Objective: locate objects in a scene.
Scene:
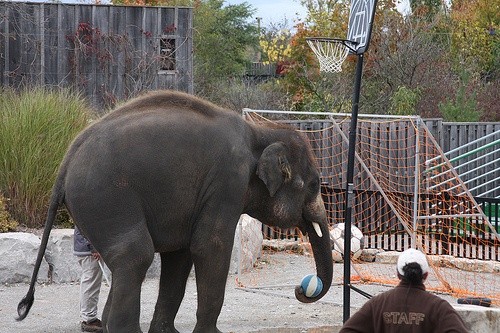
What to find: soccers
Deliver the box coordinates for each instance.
[328,221,365,263]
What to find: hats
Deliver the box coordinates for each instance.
[396,248,430,277]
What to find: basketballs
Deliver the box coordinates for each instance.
[301,275,323,298]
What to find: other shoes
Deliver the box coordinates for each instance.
[81,318,104,333]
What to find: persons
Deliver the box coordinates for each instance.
[73,225,104,333]
[339,249,470,333]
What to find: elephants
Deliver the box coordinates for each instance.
[17,91,333,333]
